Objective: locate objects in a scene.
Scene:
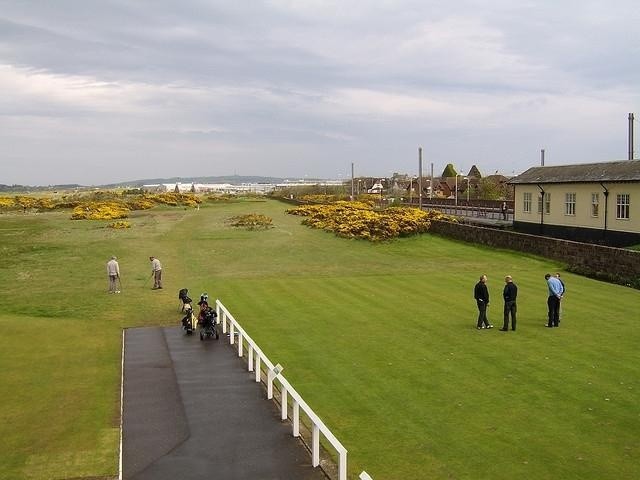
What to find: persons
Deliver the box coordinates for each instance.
[149,256,162,290]
[474,274,494,330]
[106,256,120,293]
[499,275,517,332]
[501,201,509,220]
[544,274,563,328]
[553,273,565,325]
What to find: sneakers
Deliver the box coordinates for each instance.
[486,324,493,329]
[545,323,549,327]
[499,328,506,331]
[478,326,485,329]
[151,287,162,290]
[114,291,120,294]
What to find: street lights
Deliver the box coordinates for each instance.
[357,177,393,202]
[411,176,417,207]
[464,177,469,207]
[426,179,431,204]
[455,173,463,214]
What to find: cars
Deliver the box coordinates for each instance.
[382,196,407,205]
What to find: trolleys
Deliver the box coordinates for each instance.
[200,307,220,340]
